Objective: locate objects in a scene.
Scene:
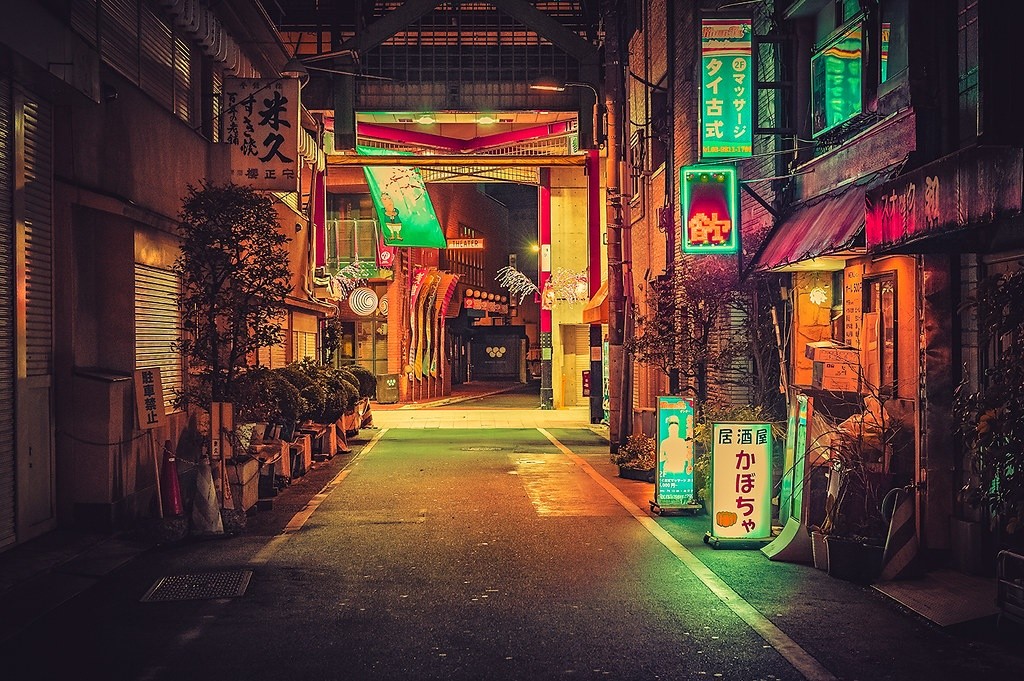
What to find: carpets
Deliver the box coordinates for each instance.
[871,568,1001,627]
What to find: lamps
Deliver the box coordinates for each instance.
[282,54,307,80]
[101,81,121,102]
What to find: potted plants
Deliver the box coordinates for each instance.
[172,355,378,512]
[610,434,656,482]
[809,355,920,578]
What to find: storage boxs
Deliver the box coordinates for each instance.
[811,361,859,392]
[805,339,860,364]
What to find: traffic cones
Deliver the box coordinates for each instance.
[879,486,927,581]
[160,438,184,515]
[188,455,226,538]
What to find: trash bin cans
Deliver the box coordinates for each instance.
[376,374,399,404]
